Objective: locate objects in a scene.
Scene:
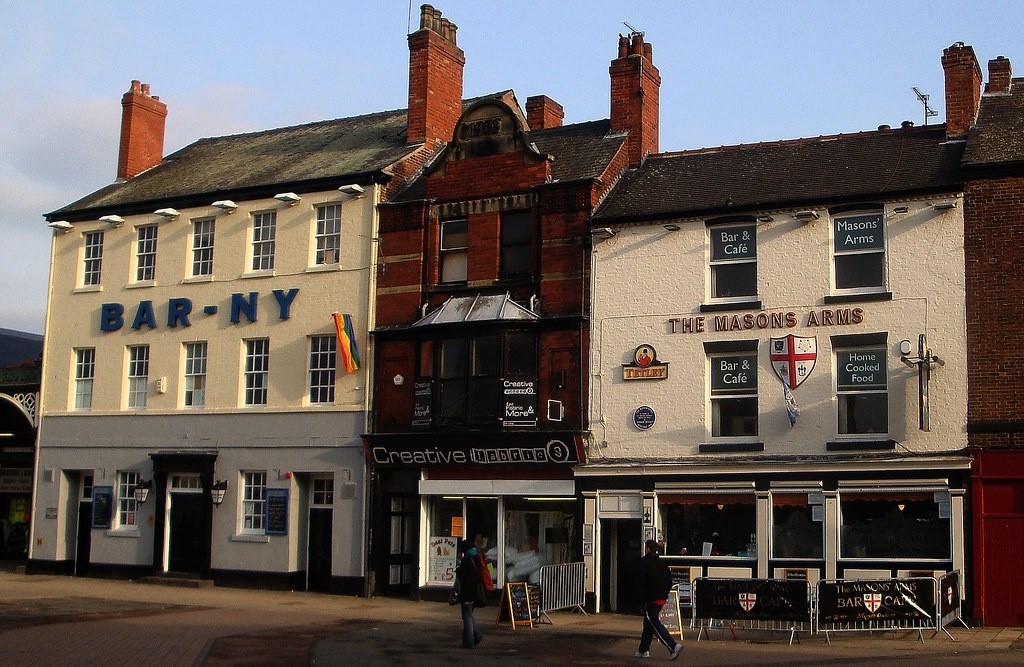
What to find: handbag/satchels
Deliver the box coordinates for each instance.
[478,553,495,590]
[448,578,461,605]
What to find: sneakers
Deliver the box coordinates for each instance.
[670,643,683,661]
[635,650,650,658]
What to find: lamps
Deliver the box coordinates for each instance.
[273,193,301,201]
[154,208,181,218]
[935,201,957,209]
[212,200,239,209]
[663,224,679,231]
[338,184,365,194]
[134,479,152,506]
[48,221,73,229]
[99,214,124,224]
[757,214,772,222]
[209,479,228,508]
[795,211,819,222]
[591,227,614,239]
[894,206,908,213]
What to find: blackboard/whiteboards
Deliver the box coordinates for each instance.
[506,582,532,623]
[667,566,693,605]
[657,590,683,635]
[497,584,513,627]
[784,568,808,584]
[909,570,935,605]
[527,585,540,618]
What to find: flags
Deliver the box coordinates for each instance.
[333,312,361,373]
[781,375,800,428]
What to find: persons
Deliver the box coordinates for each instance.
[633,539,684,661]
[453,539,485,648]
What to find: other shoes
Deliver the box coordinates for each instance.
[475,636,483,645]
